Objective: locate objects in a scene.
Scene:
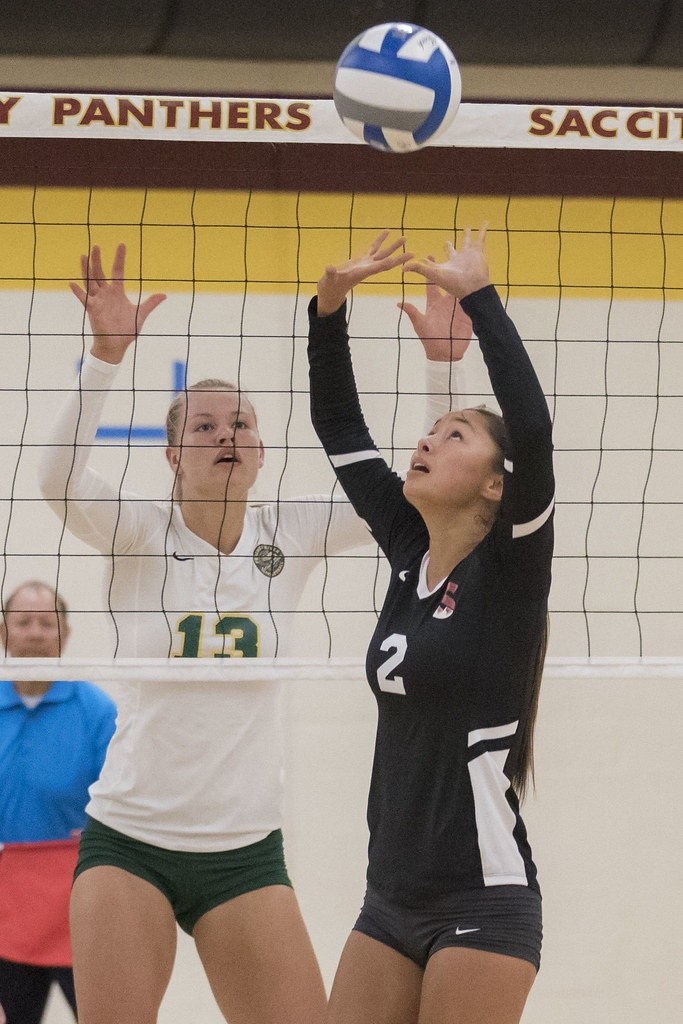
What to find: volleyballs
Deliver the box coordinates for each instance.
[333,20,463,156]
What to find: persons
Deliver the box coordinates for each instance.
[305,225,557,1023]
[36,243,484,1024]
[0,579,120,1024]
